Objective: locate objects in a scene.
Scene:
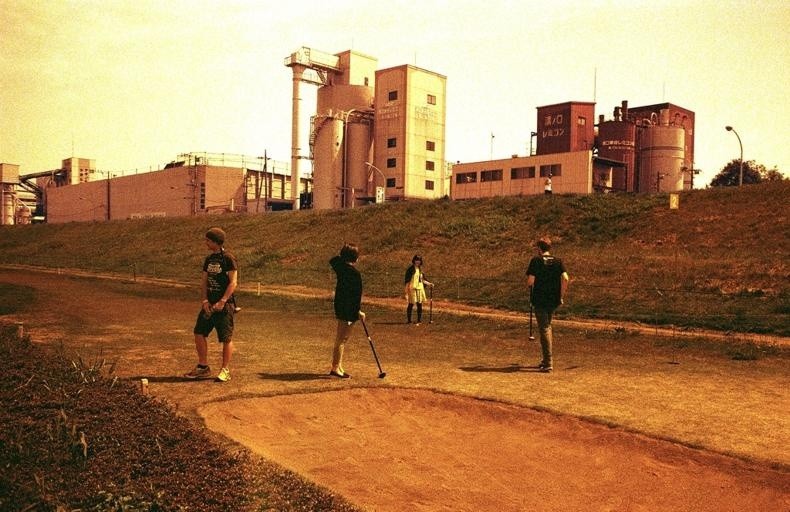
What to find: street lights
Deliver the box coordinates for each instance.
[79,195,97,222]
[364,162,387,203]
[726,125,744,187]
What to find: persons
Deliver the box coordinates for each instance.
[328,240,366,381]
[403,254,435,325]
[183,227,238,382]
[544,173,553,194]
[524,234,570,372]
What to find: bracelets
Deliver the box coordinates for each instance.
[220,297,227,303]
[201,299,209,303]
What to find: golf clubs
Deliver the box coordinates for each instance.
[221,247,241,313]
[359,317,384,377]
[527,286,534,340]
[429,288,433,323]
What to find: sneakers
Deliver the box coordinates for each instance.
[540,363,553,371]
[184,365,213,380]
[329,370,351,380]
[343,372,351,376]
[215,367,232,382]
